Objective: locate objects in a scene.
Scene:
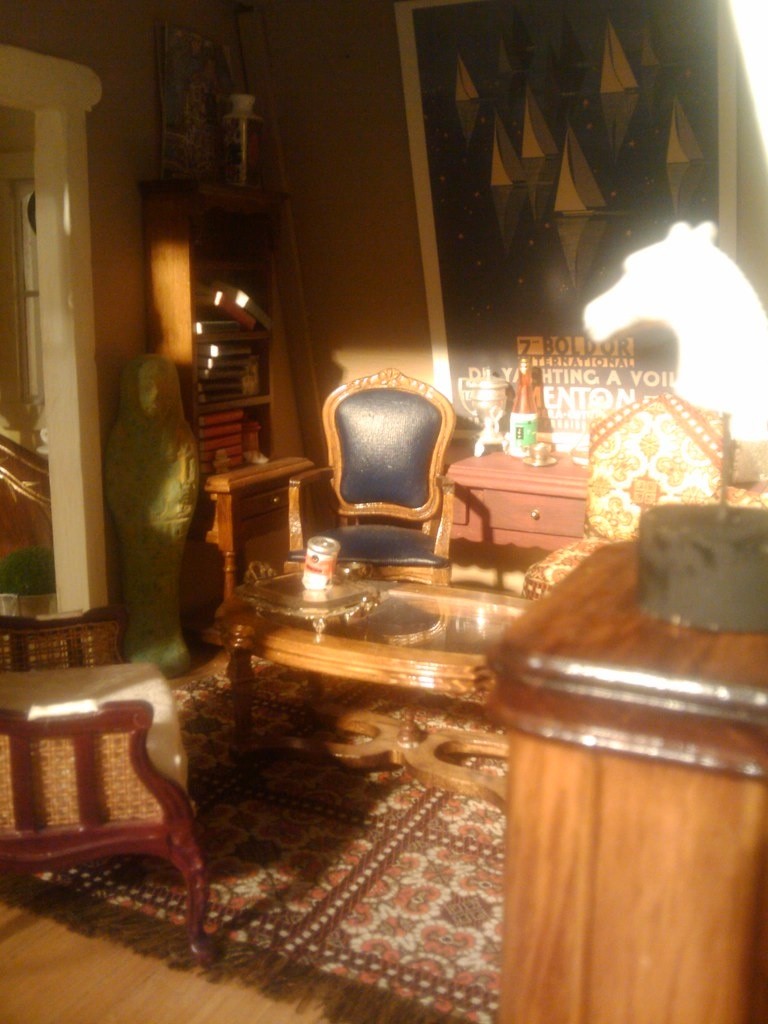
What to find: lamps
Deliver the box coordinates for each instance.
[223,91,267,184]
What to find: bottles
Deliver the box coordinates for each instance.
[224,94,265,189]
[507,357,537,458]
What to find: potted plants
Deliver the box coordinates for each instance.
[0,543,58,619]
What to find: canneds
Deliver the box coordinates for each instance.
[301,536,341,591]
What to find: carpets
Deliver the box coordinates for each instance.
[0,638,506,1023]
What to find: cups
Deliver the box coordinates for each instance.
[531,442,552,462]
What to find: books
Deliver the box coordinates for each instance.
[188,280,272,473]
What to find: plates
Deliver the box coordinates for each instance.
[522,457,557,467]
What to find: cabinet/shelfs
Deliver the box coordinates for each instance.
[140,178,286,469]
[485,545,768,1023]
[192,456,314,598]
[446,453,590,551]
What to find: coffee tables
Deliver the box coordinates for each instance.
[220,580,535,813]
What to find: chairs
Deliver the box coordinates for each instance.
[520,395,727,600]
[0,604,219,962]
[285,368,457,584]
